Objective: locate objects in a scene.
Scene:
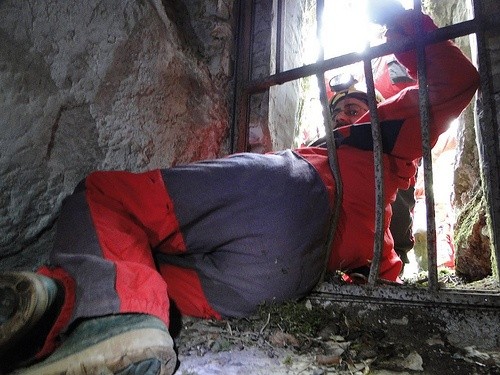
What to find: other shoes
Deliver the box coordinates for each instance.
[9,313,177,375]
[0,270,60,354]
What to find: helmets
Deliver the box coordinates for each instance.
[331,83,385,109]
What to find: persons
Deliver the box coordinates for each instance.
[321,26,423,280]
[1,1,484,375]
